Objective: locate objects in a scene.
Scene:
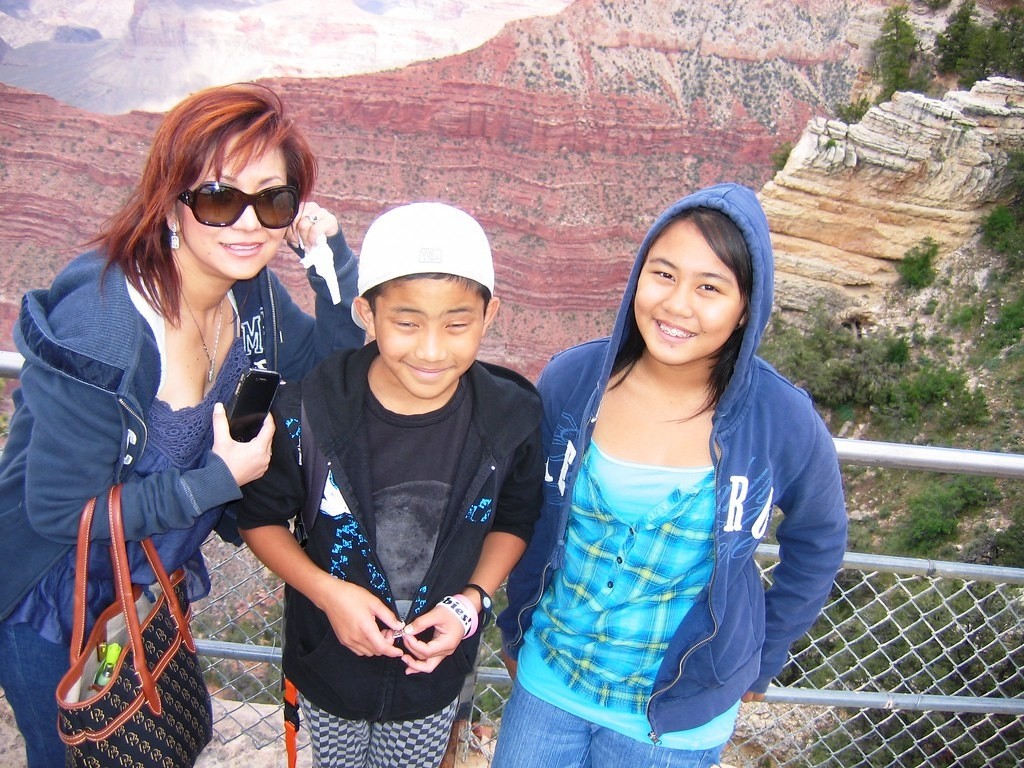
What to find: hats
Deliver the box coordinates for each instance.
[351,203,495,331]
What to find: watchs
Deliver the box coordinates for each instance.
[465,584,493,635]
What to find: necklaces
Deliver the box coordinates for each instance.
[178,284,224,382]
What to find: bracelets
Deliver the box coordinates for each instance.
[437,594,478,639]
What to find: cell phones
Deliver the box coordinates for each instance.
[226,369,282,442]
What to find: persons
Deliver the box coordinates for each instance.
[232,204,544,768]
[491,183,847,768]
[0,82,365,768]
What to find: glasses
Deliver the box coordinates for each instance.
[177,176,301,229]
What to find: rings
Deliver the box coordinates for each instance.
[302,213,319,223]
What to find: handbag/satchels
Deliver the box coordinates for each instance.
[56,484,213,768]
